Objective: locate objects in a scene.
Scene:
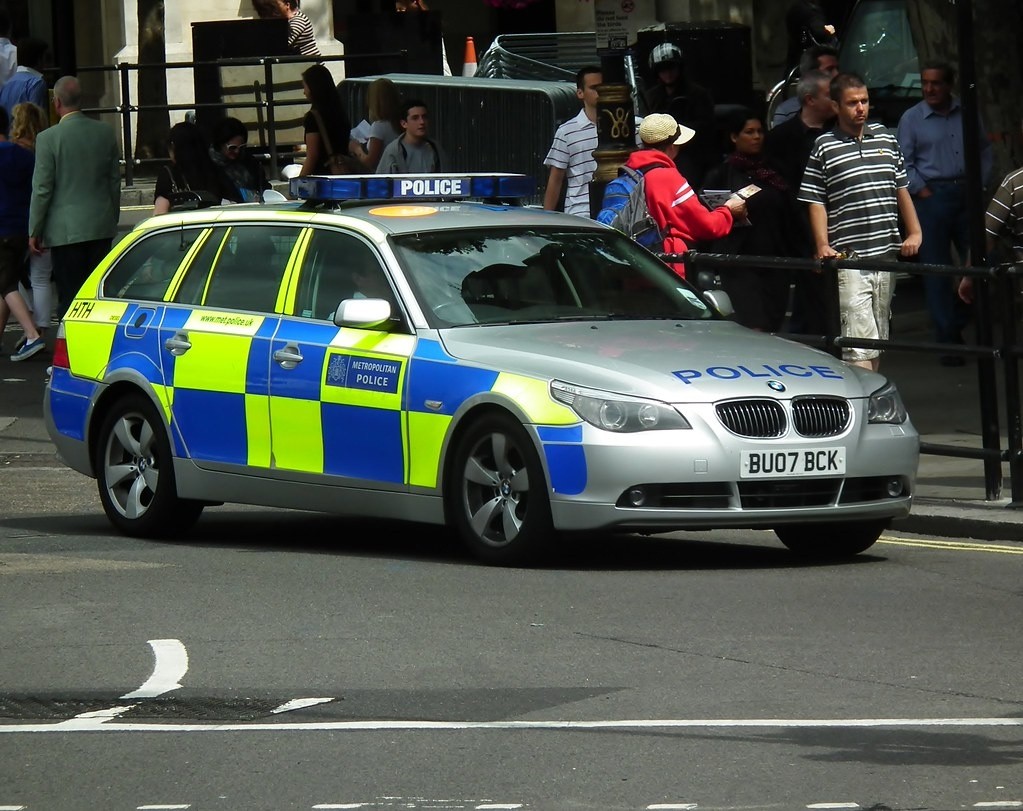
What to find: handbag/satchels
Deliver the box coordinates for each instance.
[329,154,374,175]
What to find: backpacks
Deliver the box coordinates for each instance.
[596,164,671,253]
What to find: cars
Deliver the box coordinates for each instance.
[39,174,922,570]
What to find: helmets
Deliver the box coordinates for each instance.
[647,42,685,70]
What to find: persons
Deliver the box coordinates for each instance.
[0,106,46,362]
[958,167,1023,304]
[697,44,839,357]
[10,103,58,355]
[0,8,49,128]
[153,117,272,215]
[375,99,448,174]
[625,113,748,281]
[796,73,922,372]
[396,0,428,11]
[895,60,996,367]
[28,76,121,375]
[286,65,351,200]
[325,245,395,331]
[251,0,325,65]
[348,78,407,175]
[639,42,723,192]
[543,68,644,221]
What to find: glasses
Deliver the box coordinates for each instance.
[225,143,247,151]
[51,97,59,103]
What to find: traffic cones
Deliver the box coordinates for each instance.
[462,35,478,78]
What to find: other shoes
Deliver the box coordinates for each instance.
[10,337,45,361]
[941,337,965,366]
[15,328,41,349]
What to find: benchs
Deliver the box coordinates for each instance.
[134,258,275,309]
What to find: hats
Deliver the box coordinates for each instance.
[640,112,696,149]
